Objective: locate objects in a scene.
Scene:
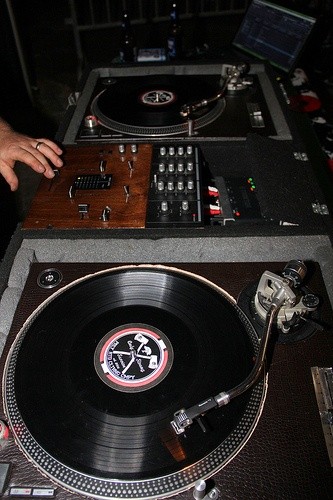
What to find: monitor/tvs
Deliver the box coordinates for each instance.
[230,0,316,73]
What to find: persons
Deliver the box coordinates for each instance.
[0,118,64,192]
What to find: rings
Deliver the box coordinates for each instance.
[36,142,43,150]
